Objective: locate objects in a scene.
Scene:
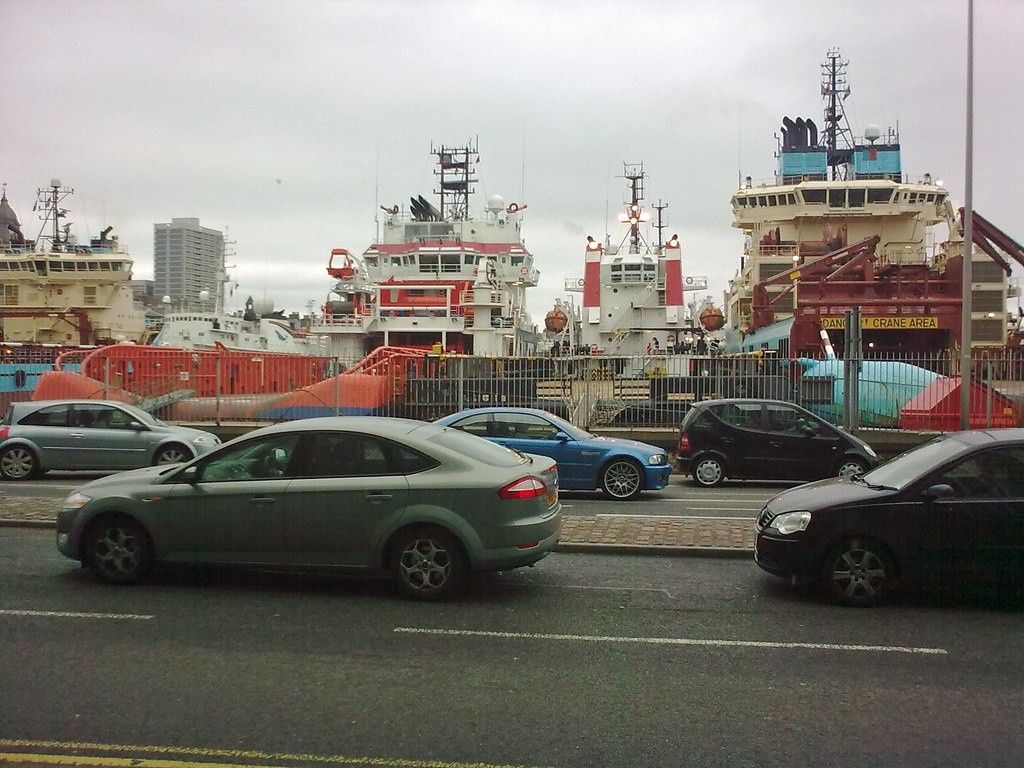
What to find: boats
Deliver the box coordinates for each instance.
[0,181,323,402]
[546,310,568,331]
[309,135,541,374]
[715,46,1024,386]
[323,249,355,278]
[699,304,724,332]
[541,162,725,398]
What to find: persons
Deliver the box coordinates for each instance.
[96,409,113,428]
[653,337,660,350]
[647,343,651,356]
[514,418,532,439]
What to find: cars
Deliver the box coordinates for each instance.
[57,417,562,599]
[0,397,226,479]
[675,398,881,486]
[429,407,673,501]
[752,426,1024,608]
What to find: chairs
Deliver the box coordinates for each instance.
[379,443,414,473]
[332,437,363,475]
[500,421,510,436]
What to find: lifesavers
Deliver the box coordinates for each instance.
[393,205,399,212]
[67,334,72,339]
[15,370,26,386]
[590,343,598,350]
[510,203,518,212]
[578,278,584,285]
[56,288,62,294]
[520,267,528,275]
[667,335,674,341]
[686,276,693,284]
